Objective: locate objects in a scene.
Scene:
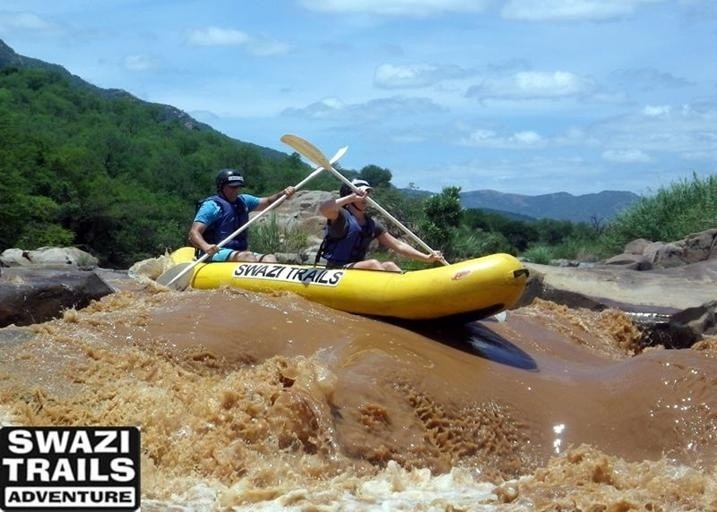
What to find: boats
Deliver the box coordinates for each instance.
[168,244,534,325]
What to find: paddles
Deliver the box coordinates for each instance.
[155,146,350,291]
[281,134,451,267]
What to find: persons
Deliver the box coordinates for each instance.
[313,178,445,274]
[187,168,295,262]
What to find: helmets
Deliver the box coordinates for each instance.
[216,168,246,190]
[341,177,374,197]
